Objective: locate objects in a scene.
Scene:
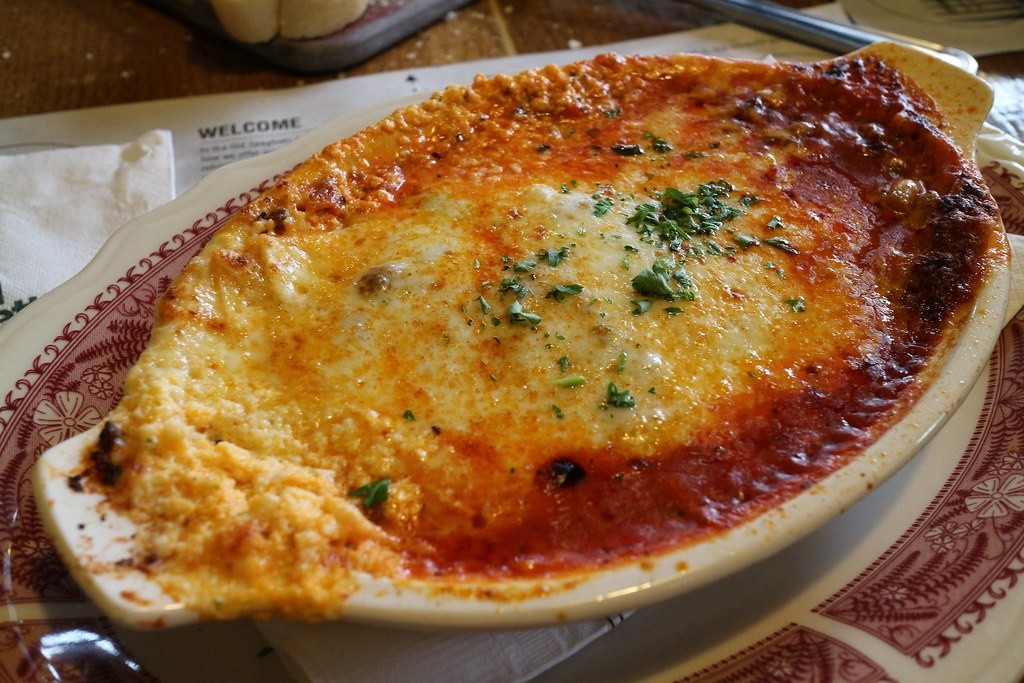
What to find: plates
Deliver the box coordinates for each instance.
[0,100,1024,683]
[32,41,1010,633]
